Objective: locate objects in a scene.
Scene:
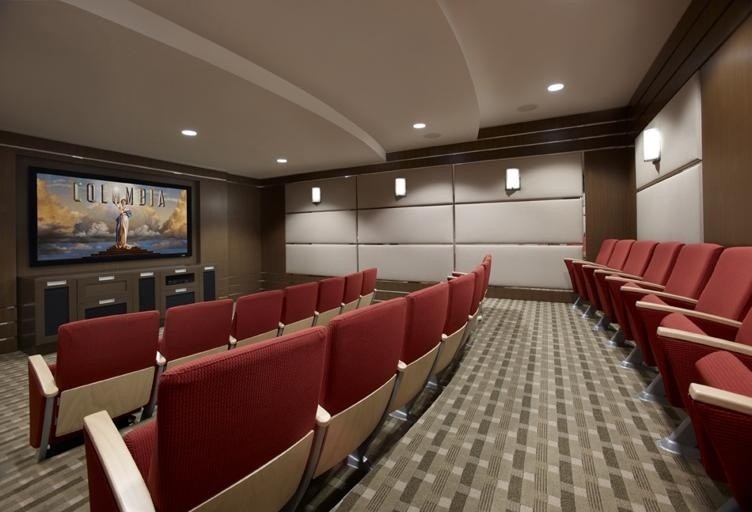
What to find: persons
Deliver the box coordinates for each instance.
[111,196,135,250]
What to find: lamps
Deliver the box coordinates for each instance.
[642,128,662,162]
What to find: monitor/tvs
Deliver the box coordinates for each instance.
[27,167,192,267]
